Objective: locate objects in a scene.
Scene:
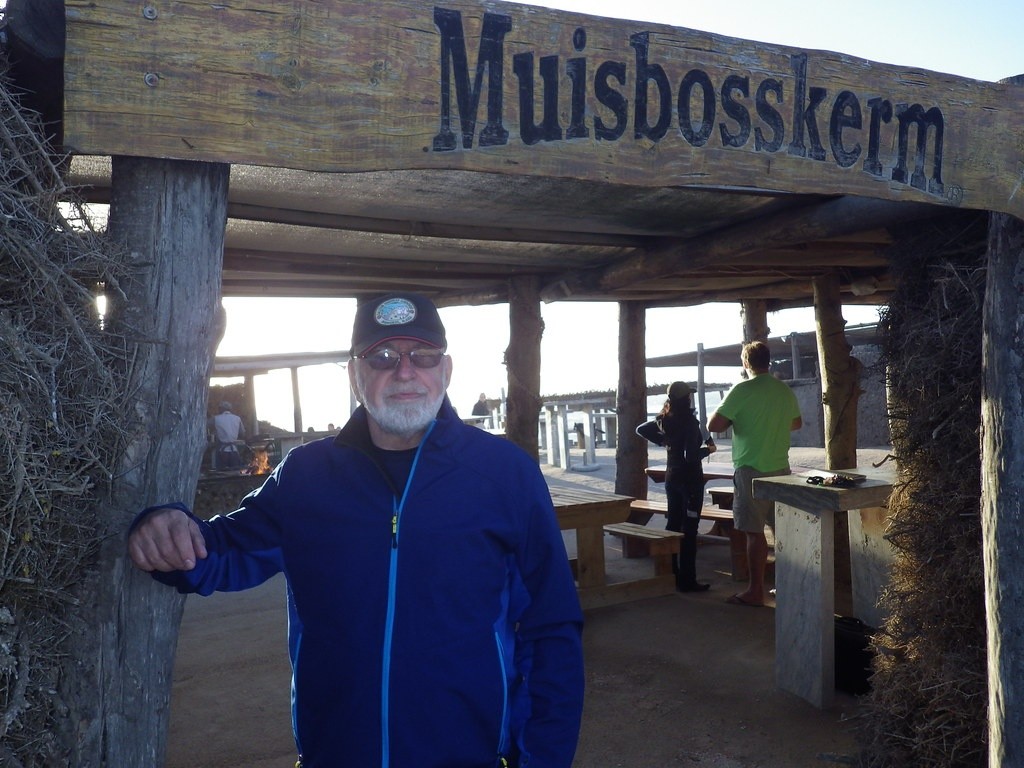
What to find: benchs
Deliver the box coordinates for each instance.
[602,523,684,577]
[704,485,734,537]
[628,500,749,581]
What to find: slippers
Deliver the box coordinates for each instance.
[726,593,766,606]
[769,589,776,598]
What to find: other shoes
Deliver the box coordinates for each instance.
[672,568,678,576]
[677,583,710,593]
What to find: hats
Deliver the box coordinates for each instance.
[669,382,697,401]
[351,291,447,359]
[223,402,232,411]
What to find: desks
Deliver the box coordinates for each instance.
[644,464,775,576]
[751,465,895,710]
[549,483,675,611]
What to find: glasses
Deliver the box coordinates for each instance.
[352,347,444,371]
[806,476,826,485]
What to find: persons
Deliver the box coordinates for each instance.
[472,393,489,429]
[307,427,314,432]
[635,382,716,593]
[206,401,245,471]
[328,423,338,431]
[706,340,803,604]
[128,292,583,768]
